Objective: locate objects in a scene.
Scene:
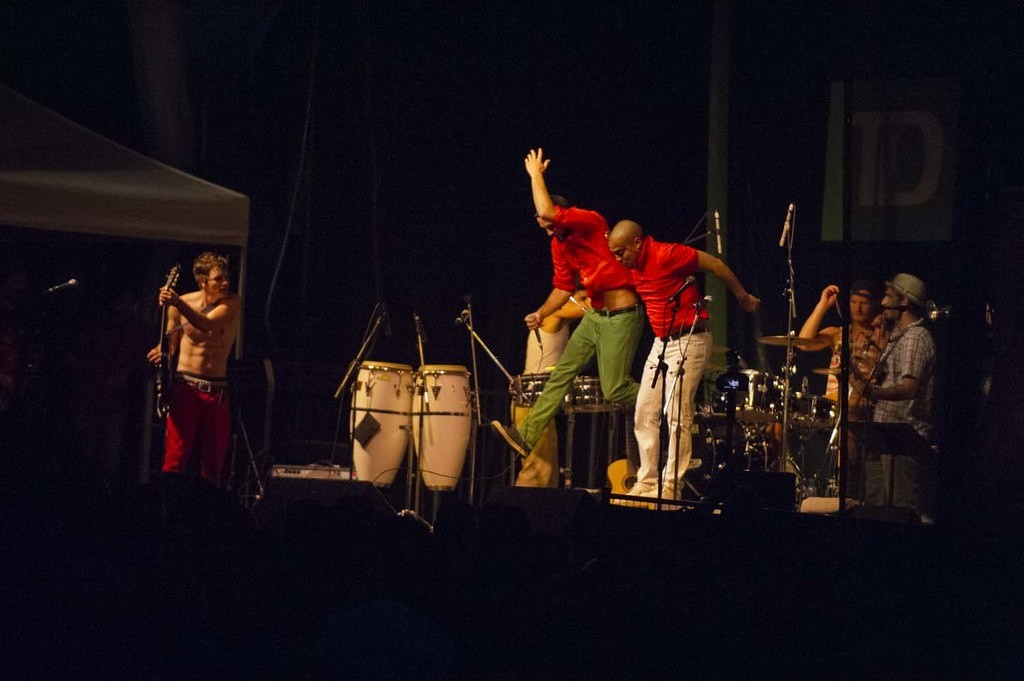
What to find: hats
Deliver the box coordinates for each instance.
[850,281,877,299]
[885,272,930,308]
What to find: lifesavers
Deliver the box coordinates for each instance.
[509,373,552,407]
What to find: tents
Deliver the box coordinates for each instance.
[0,85,250,357]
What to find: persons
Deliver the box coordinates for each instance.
[510,288,589,490]
[796,273,890,501]
[147,253,240,488]
[490,147,642,456]
[850,273,937,519]
[0,398,1024,681]
[606,219,760,500]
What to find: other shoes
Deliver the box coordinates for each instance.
[490,419,530,460]
[614,483,654,503]
[640,485,683,501]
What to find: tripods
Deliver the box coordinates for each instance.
[759,232,815,497]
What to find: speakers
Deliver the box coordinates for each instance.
[716,470,798,516]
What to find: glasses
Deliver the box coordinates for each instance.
[199,272,231,282]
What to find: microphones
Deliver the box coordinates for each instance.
[415,312,429,342]
[714,211,722,255]
[779,203,793,247]
[529,315,543,349]
[380,304,394,337]
[48,277,77,293]
[669,276,696,301]
[454,309,468,325]
[693,294,714,308]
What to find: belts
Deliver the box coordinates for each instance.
[595,303,643,316]
[176,374,230,393]
[661,326,711,343]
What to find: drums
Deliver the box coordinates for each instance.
[745,420,783,458]
[730,364,786,423]
[410,365,472,491]
[789,392,834,429]
[562,377,621,415]
[349,361,415,487]
[704,409,738,446]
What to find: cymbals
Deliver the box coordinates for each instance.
[706,363,722,371]
[758,334,825,346]
[713,345,730,353]
[812,369,841,375]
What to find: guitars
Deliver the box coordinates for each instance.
[156,265,180,419]
